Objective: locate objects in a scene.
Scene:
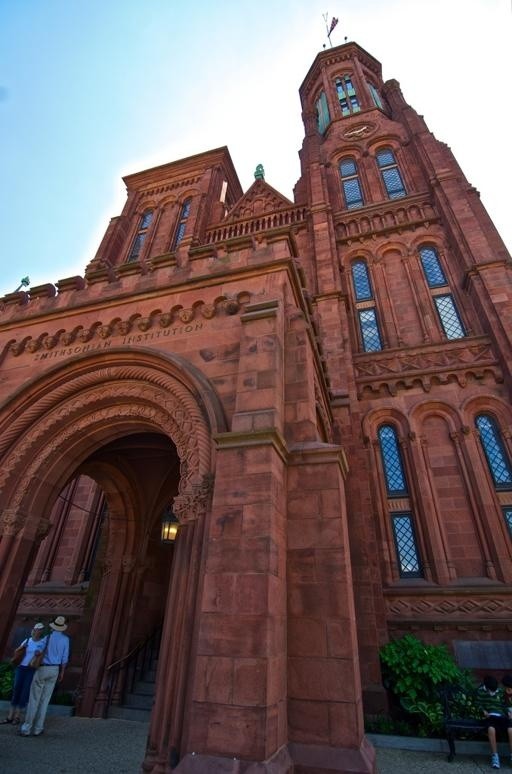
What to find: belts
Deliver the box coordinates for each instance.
[40,663,60,666]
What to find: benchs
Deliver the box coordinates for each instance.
[441,686,510,763]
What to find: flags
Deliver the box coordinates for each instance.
[328,15,339,37]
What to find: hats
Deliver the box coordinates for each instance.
[49,614,69,631]
[34,622,45,630]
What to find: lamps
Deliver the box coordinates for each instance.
[160,507,180,544]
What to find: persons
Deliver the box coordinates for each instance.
[0,621,46,725]
[500,673,511,722]
[15,615,70,737]
[473,673,512,769]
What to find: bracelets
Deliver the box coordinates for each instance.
[61,669,65,672]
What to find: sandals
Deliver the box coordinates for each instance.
[0,716,45,737]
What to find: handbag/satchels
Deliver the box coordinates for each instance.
[10,637,30,666]
[29,647,46,670]
